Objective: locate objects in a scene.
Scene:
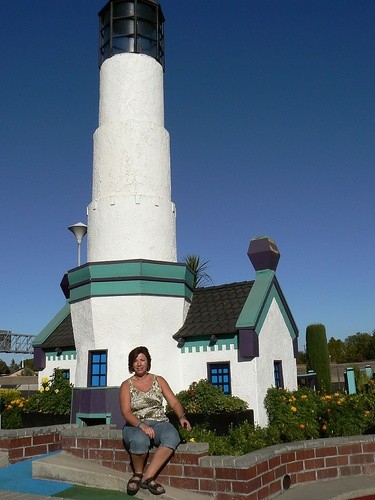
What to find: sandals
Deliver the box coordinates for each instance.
[126,473,143,496]
[139,476,165,495]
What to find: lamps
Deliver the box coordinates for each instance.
[68,221,88,267]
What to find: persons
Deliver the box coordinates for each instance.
[119,347,192,495]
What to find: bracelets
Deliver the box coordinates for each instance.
[137,421,144,428]
[177,415,187,422]
